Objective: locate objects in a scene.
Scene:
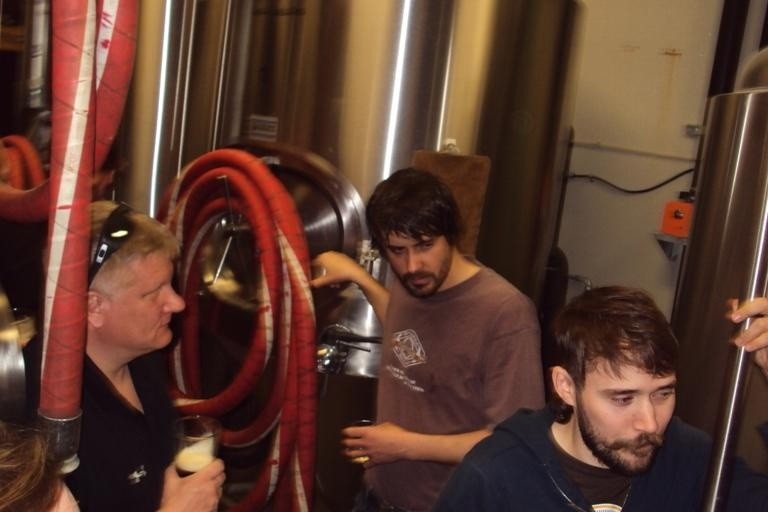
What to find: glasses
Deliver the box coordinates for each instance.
[89,200,136,288]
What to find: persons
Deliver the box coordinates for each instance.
[308,170,547,512]
[64,199,227,512]
[0,422,81,512]
[434,286,768,512]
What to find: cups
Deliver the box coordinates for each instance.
[175,415,218,477]
